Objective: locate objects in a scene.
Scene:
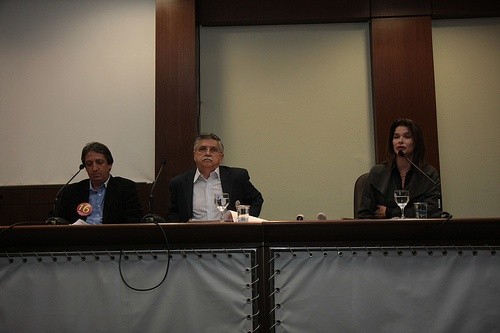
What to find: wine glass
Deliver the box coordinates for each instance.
[214,194,229,223]
[394,190,409,219]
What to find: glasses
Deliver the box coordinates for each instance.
[198,145,220,154]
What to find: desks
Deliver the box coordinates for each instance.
[263,217,500,333]
[0,222,265,333]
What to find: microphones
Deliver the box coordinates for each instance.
[46,163,84,225]
[398,150,438,186]
[141,159,167,223]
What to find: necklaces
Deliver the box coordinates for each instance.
[401,175,406,177]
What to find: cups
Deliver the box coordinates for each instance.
[236,205,250,222]
[413,203,427,219]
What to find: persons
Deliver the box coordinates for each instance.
[357,118,442,218]
[46,142,143,224]
[165,132,264,223]
